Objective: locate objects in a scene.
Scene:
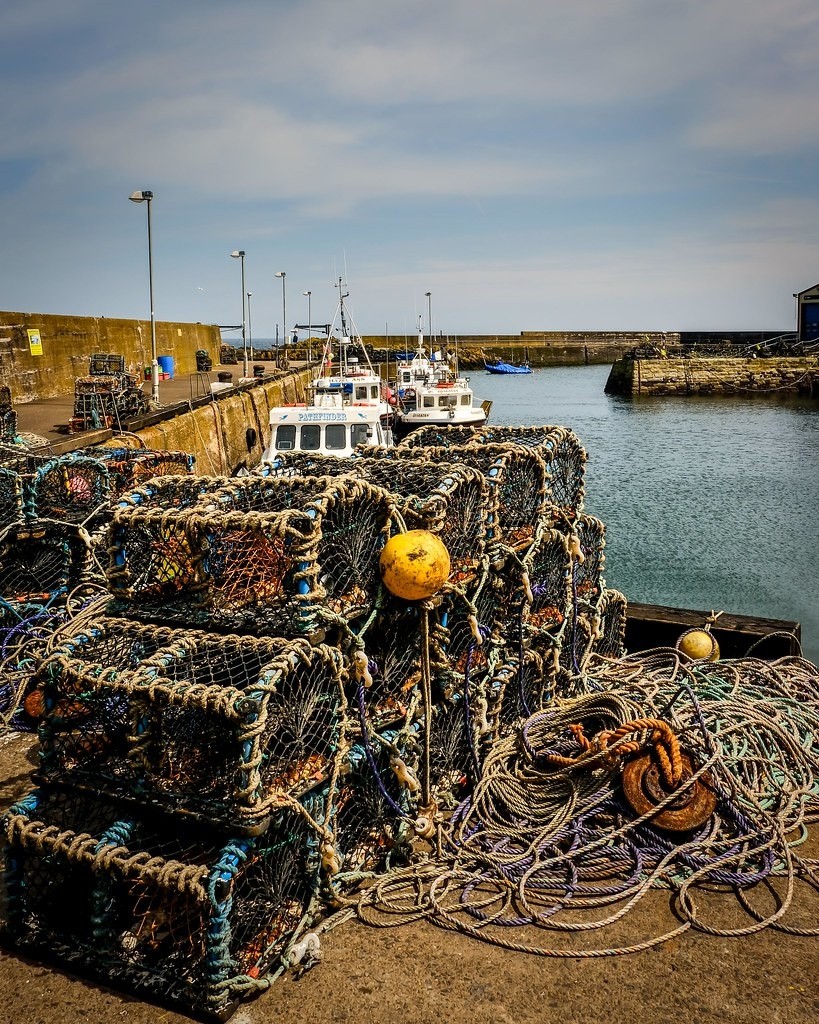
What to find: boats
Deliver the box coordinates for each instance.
[236,252,494,477]
[480,350,534,374]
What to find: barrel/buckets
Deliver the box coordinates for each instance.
[157,355,174,379]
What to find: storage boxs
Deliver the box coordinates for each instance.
[164,373,170,380]
[145,375,150,380]
[149,374,163,380]
[145,366,163,375]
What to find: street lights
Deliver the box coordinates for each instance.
[301,291,312,362]
[246,291,253,362]
[230,250,249,378]
[127,190,160,407]
[275,271,287,360]
[425,293,433,359]
